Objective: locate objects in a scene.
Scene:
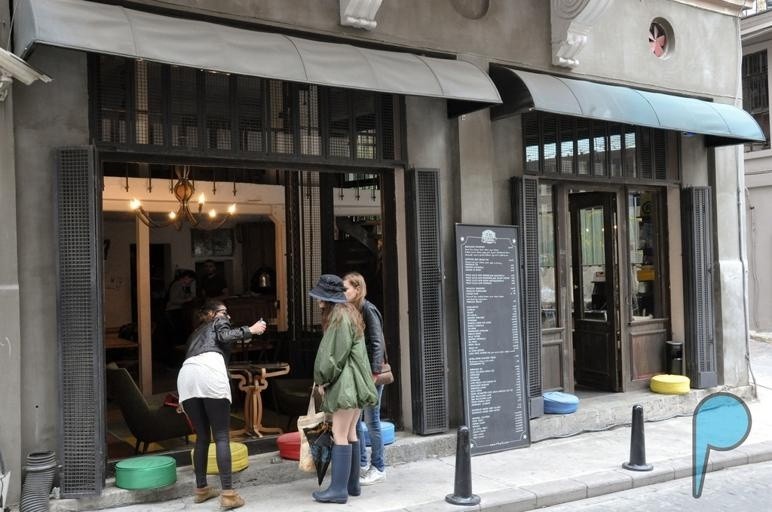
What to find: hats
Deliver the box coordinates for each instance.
[308,274,347,303]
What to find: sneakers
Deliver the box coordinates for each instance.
[359,465,385,486]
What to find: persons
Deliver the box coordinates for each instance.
[176,296,267,511]
[310,274,380,505]
[340,271,396,486]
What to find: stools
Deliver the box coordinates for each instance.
[650,374,692,395]
[275,432,306,461]
[190,442,248,474]
[361,421,396,446]
[542,392,579,413]
[115,455,176,490]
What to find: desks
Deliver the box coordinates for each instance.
[226,360,290,440]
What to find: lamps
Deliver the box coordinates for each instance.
[129,164,237,231]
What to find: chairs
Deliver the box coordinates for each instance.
[271,376,318,413]
[106,361,187,455]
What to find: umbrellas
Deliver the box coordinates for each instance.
[303,422,335,487]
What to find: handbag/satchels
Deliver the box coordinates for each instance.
[374,364,394,385]
[297,410,326,474]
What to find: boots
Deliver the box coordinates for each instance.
[312,443,352,504]
[220,489,244,508]
[349,439,362,496]
[194,486,218,504]
